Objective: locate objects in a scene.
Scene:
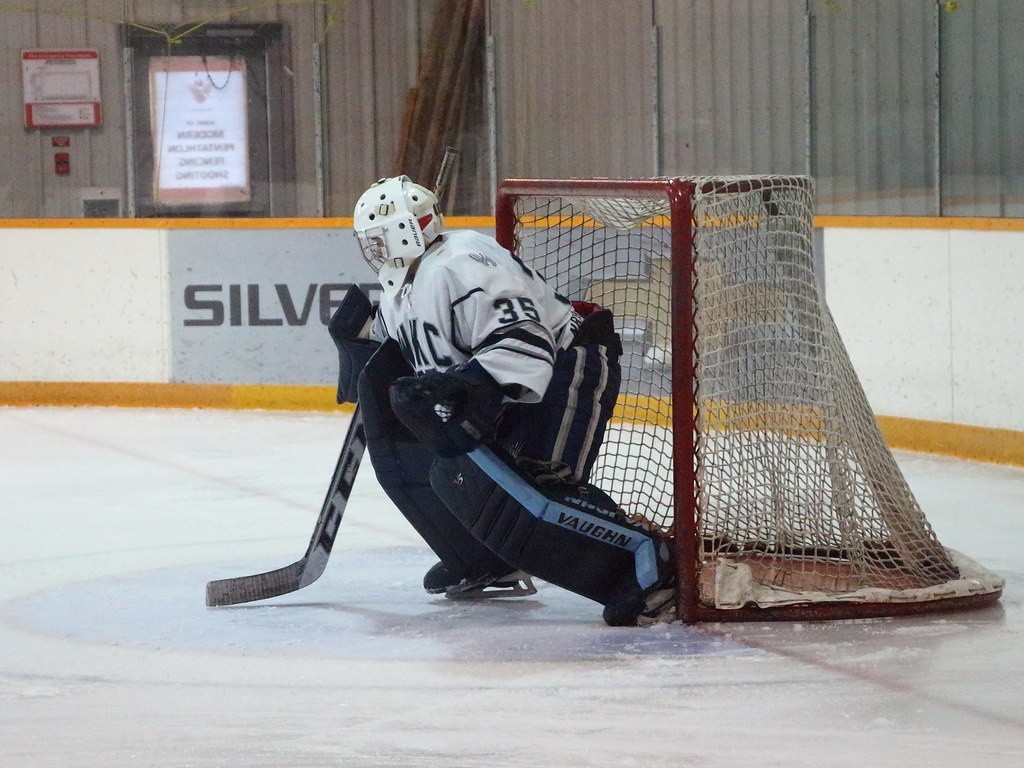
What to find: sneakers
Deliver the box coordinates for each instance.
[603,537,677,627]
[424,561,538,597]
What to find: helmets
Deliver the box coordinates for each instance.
[354,175,444,300]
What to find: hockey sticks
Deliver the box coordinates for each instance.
[205,146,460,608]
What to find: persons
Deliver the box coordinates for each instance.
[352,174,678,626]
